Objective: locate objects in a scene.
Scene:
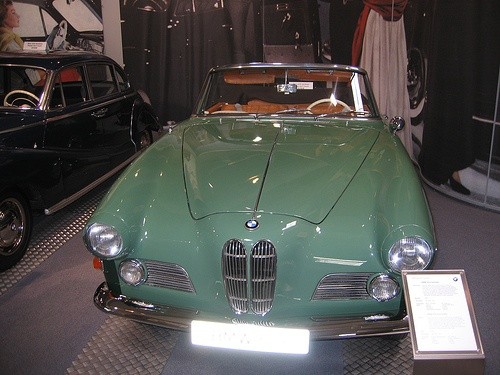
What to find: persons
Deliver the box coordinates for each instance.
[0,0,24,52]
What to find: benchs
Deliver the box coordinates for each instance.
[216,96,371,118]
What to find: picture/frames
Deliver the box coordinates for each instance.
[401,269,486,361]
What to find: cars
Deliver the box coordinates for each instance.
[1,49,168,275]
[84,62,437,341]
